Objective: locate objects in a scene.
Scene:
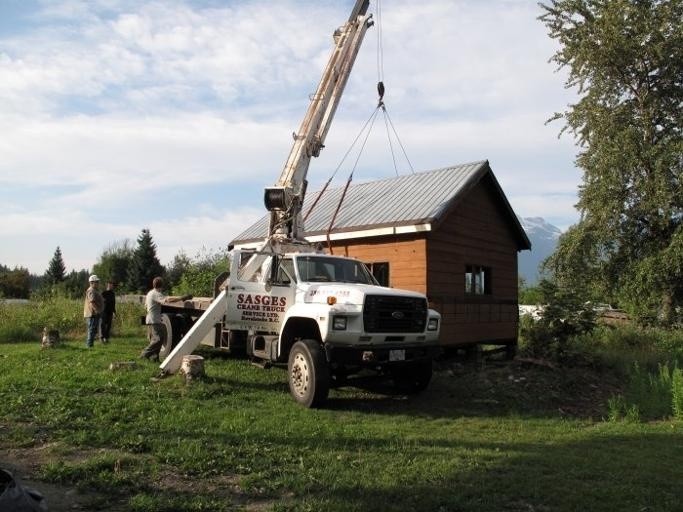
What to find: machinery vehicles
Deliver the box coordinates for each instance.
[138,0,441,412]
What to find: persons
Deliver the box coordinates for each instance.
[84,275,105,347]
[99,282,115,342]
[139,276,193,362]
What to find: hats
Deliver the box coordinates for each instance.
[89,275,99,282]
